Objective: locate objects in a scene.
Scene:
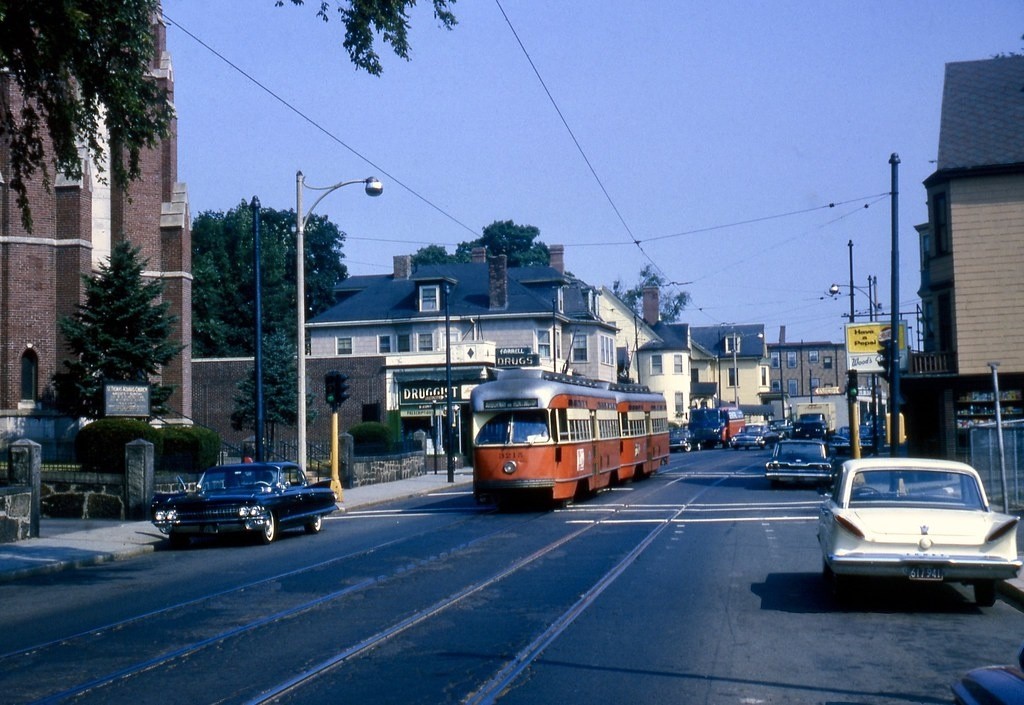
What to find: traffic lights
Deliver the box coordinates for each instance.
[339,376,351,406]
[846,371,859,396]
[324,373,339,405]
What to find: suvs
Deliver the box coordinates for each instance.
[764,420,790,445]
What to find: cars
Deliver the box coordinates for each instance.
[948,646,1024,705]
[818,456,1020,607]
[153,462,336,546]
[670,429,701,450]
[730,424,770,450]
[765,440,833,488]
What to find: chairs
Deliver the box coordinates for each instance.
[255,471,273,487]
[224,473,247,488]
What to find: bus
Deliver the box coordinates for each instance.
[469,369,669,507]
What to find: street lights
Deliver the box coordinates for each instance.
[249,193,269,461]
[732,331,764,406]
[293,170,383,481]
[442,285,457,482]
[885,152,902,455]
[829,276,884,454]
[847,239,856,450]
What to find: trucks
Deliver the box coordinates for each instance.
[689,406,745,449]
[794,403,835,439]
